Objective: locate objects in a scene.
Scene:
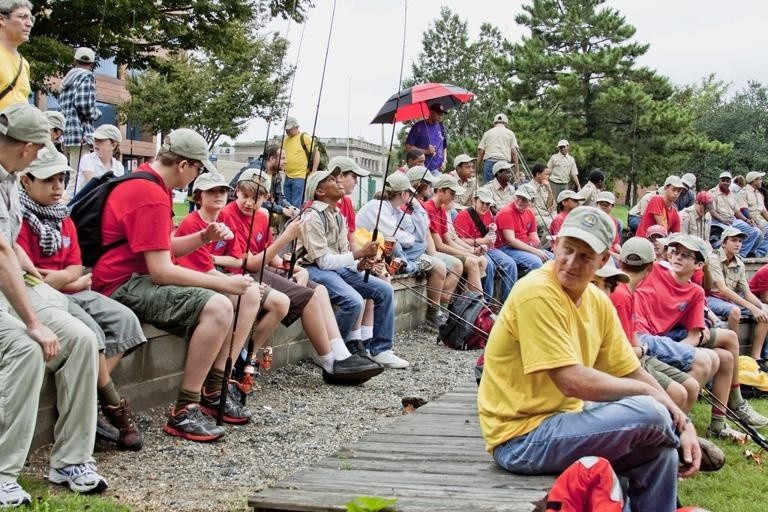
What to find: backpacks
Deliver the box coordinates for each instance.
[65,170,175,267]
[300,132,330,171]
[437,290,495,351]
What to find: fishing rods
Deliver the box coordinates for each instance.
[216,2,337,427]
[697,142,707,240]
[129,2,134,172]
[379,79,477,260]
[500,142,559,245]
[394,212,768,458]
[364,2,411,282]
[70,2,109,200]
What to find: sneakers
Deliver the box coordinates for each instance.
[49,462,109,493]
[162,404,225,440]
[197,385,252,421]
[306,338,409,385]
[0,481,32,508]
[706,422,752,446]
[722,401,768,430]
[681,438,725,471]
[424,309,448,329]
[95,398,143,452]
[414,258,434,272]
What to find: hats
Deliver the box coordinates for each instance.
[162,127,219,177]
[665,173,696,190]
[0,102,77,180]
[514,183,536,201]
[746,171,765,182]
[191,169,273,194]
[303,156,372,201]
[594,255,631,284]
[696,191,715,211]
[471,187,496,204]
[556,190,616,254]
[453,154,478,169]
[386,165,467,195]
[721,228,747,244]
[285,116,299,130]
[429,103,447,115]
[719,172,732,179]
[494,114,508,124]
[74,47,95,62]
[619,225,709,266]
[556,140,569,148]
[85,124,122,143]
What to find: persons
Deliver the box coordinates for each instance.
[58,47,102,197]
[0,0,36,111]
[0,103,768,512]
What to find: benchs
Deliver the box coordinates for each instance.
[252,378,561,512]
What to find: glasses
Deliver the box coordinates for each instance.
[7,12,36,24]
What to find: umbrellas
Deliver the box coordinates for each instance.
[369,83,475,145]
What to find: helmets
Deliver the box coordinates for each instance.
[492,161,516,177]
